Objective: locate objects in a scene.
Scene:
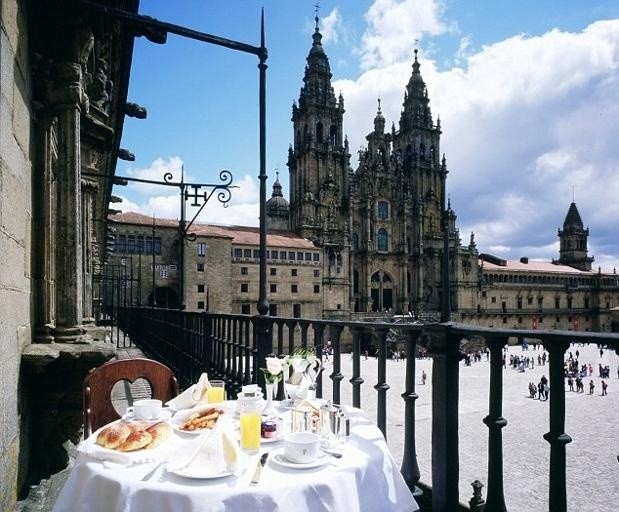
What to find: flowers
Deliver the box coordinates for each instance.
[258,343,325,384]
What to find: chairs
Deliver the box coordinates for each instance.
[82,357,180,440]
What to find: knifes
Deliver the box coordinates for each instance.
[250,453,268,484]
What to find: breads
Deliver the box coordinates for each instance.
[97,419,170,452]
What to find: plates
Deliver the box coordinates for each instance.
[270,447,329,468]
[123,409,171,422]
[76,420,181,462]
[174,404,228,434]
[170,448,247,480]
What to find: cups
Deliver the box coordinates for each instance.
[283,432,330,463]
[240,404,261,453]
[207,379,225,404]
[127,399,162,419]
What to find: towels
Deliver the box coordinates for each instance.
[296,399,328,411]
[165,424,248,476]
[165,371,211,411]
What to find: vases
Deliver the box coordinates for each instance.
[261,383,279,416]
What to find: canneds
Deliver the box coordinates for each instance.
[261,420,278,438]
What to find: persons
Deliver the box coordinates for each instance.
[322,342,334,362]
[458,338,618,400]
[421,370,426,385]
[363,351,369,360]
[389,348,432,362]
[374,348,379,359]
